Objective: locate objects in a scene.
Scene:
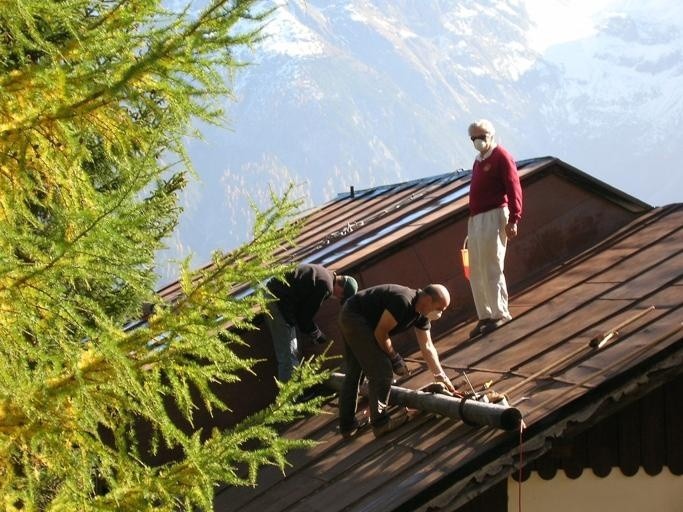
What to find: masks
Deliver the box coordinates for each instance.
[474,139,487,150]
[425,309,443,321]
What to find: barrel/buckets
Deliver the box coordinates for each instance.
[461,247,471,279]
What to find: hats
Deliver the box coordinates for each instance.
[468,121,495,136]
[340,276,358,305]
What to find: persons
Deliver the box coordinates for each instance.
[468,119,524,341]
[339,284,451,438]
[263,264,359,401]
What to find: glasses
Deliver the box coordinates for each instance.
[471,135,485,141]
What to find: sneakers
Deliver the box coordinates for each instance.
[480,317,512,334]
[373,408,408,439]
[345,415,368,441]
[470,319,490,339]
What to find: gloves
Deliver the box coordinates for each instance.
[390,353,408,376]
[313,329,328,345]
[434,373,456,394]
